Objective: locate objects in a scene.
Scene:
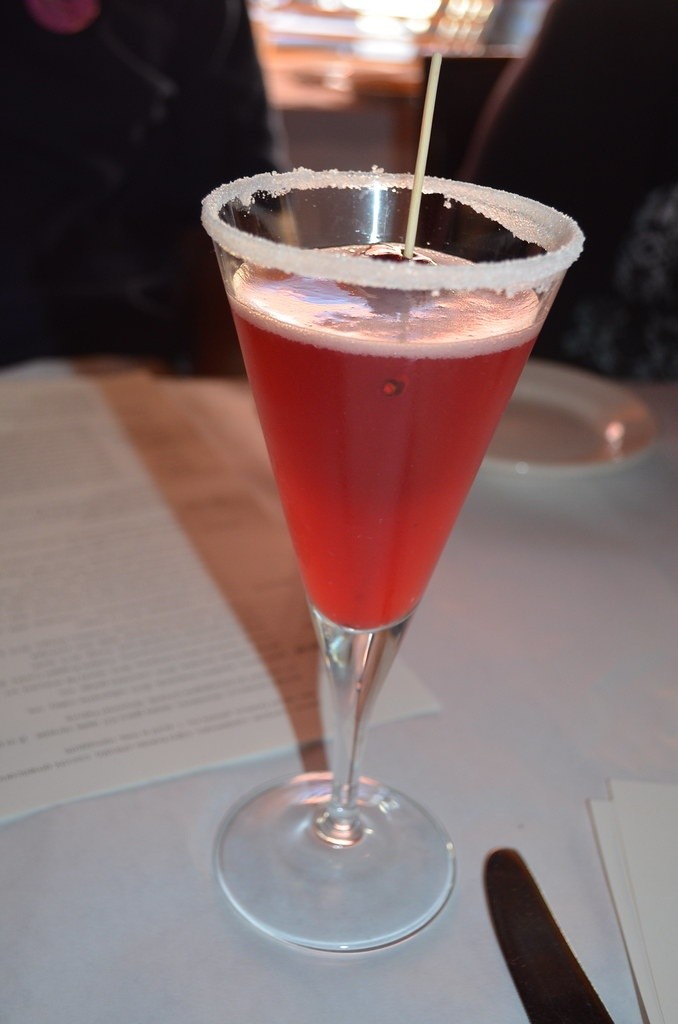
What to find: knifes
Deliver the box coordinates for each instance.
[483,848,615,1024]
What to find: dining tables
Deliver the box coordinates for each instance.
[0,340,674,1022]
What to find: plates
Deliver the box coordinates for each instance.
[481,359,658,475]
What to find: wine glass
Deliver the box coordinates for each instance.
[210,167,582,944]
